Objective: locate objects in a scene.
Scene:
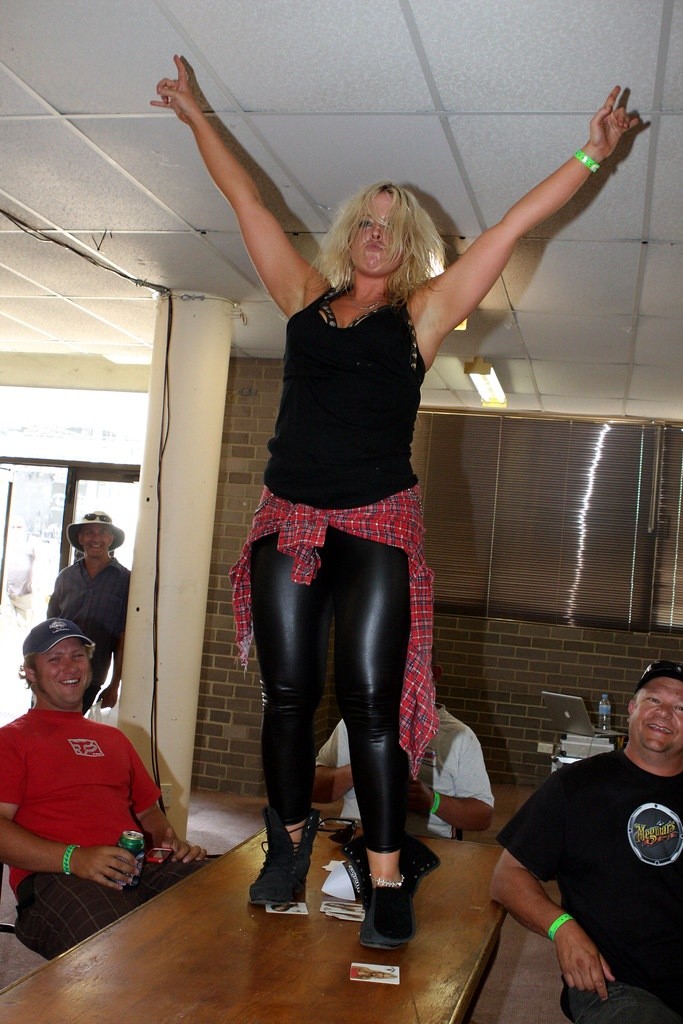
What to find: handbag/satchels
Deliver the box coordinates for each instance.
[88,696,119,728]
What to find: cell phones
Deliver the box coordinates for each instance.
[145,847,174,864]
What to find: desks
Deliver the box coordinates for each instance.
[0,824,508,1024]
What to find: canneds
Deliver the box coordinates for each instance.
[112,831,144,886]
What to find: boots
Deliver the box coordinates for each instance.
[249,805,322,905]
[337,833,442,947]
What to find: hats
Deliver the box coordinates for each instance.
[634,658,683,694]
[23,618,93,658]
[66,511,125,552]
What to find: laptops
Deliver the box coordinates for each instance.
[542,691,628,738]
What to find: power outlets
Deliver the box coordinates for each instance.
[157,783,172,808]
[537,742,554,754]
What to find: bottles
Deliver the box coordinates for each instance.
[599,694,612,732]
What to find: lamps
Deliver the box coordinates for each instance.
[464,356,508,408]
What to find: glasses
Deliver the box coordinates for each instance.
[85,514,112,522]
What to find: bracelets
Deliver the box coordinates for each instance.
[547,914,575,943]
[429,788,440,814]
[63,845,78,877]
[575,150,601,174]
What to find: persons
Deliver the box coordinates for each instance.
[0,616,218,960]
[325,902,362,913]
[311,701,495,838]
[150,54,641,948]
[48,512,131,717]
[271,903,300,914]
[494,660,683,1024]
[350,966,397,981]
